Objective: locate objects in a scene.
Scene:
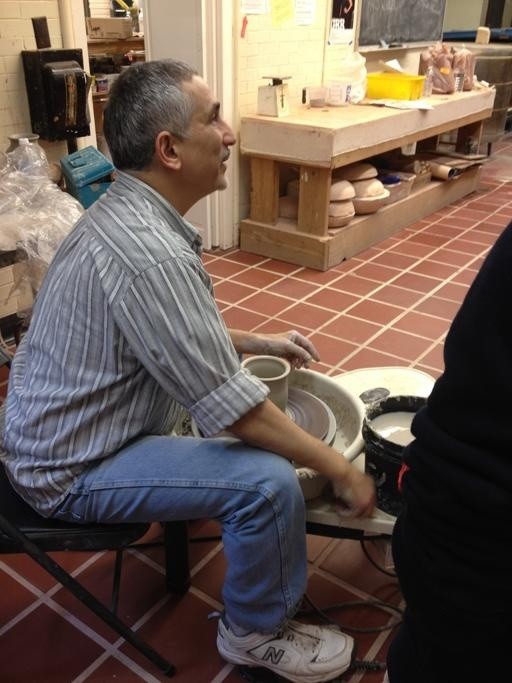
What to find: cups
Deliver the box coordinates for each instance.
[242,355,291,412]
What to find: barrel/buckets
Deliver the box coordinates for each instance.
[362,396,428,518]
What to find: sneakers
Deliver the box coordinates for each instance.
[207,609,358,683]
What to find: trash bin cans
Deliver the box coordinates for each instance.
[61,145,115,210]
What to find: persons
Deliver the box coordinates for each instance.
[385,214,512,683]
[0,59,376,683]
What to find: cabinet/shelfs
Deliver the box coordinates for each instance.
[86,35,147,141]
[238,87,499,271]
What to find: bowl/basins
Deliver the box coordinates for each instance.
[190,367,367,498]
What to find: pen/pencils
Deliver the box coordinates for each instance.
[389,45,402,47]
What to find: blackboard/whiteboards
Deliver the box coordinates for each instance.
[354,0,448,53]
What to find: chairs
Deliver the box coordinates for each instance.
[0,496,183,677]
